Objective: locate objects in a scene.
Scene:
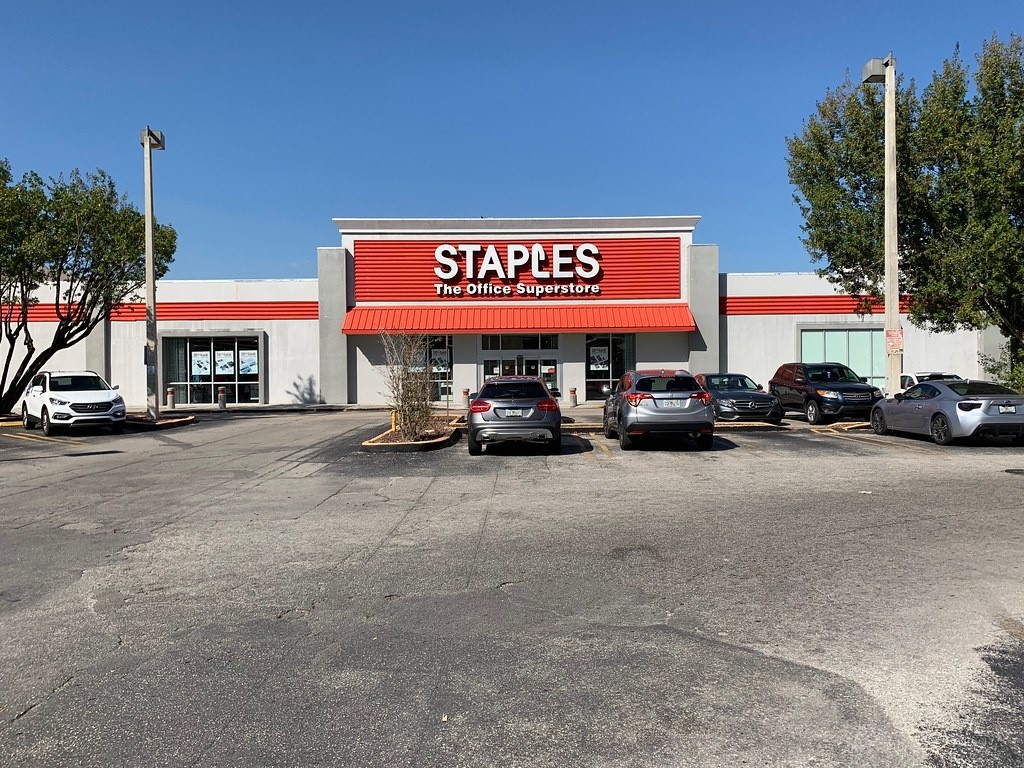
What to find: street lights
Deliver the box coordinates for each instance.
[140,127,164,420]
[860,53,906,401]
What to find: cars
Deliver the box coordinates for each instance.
[870,377,1024,445]
[694,373,783,426]
[902,372,965,397]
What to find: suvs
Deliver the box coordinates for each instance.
[769,361,884,425]
[468,375,562,454]
[23,371,128,436]
[601,369,715,449]
